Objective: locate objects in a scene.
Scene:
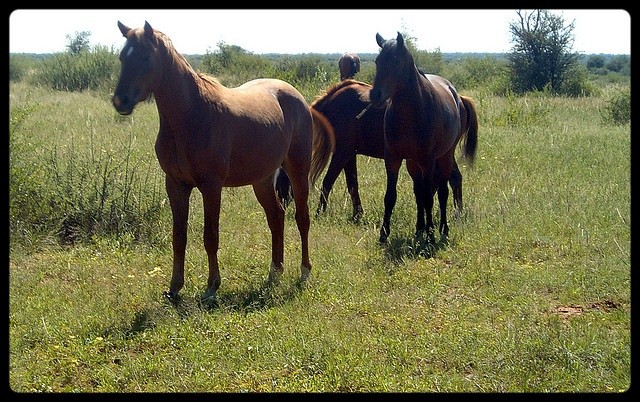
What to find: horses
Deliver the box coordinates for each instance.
[338,52,360,81]
[275,79,478,223]
[111,20,335,310]
[369,31,461,246]
